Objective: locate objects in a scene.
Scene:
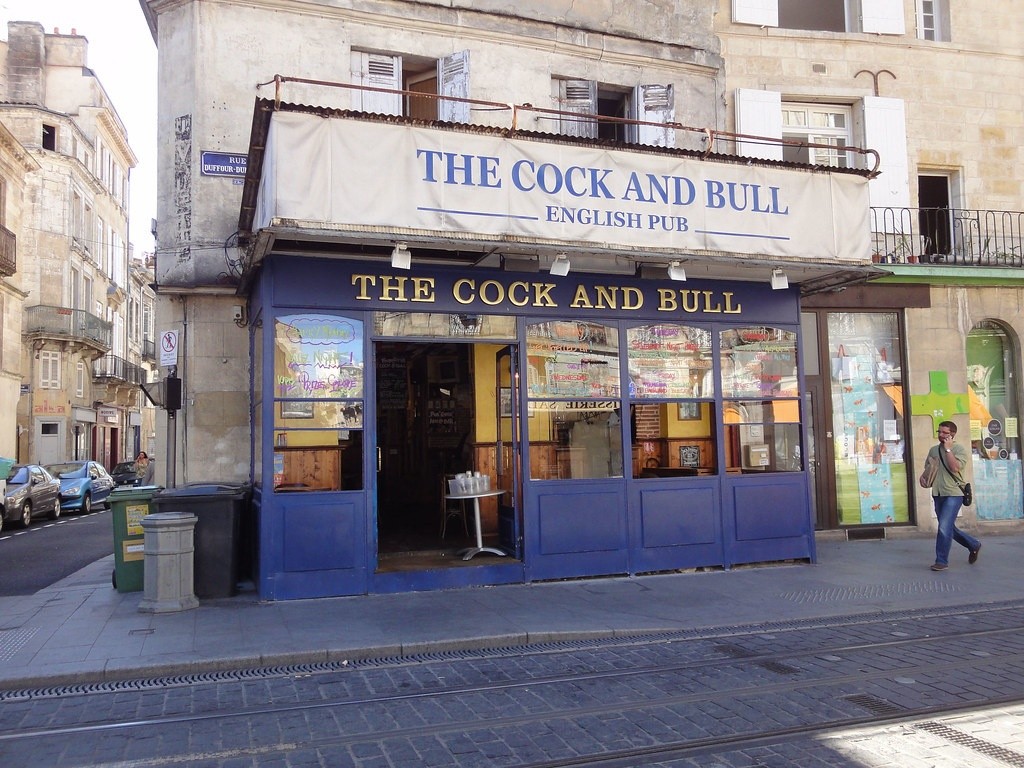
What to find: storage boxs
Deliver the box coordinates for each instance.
[448,477,490,496]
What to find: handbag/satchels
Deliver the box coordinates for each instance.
[963,483,972,507]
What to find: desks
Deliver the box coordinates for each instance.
[446,491,507,560]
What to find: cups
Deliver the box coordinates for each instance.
[455,470,486,494]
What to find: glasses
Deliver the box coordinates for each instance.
[936,430,953,434]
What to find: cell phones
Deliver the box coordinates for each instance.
[950,434,954,440]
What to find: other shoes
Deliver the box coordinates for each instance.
[968,540,981,564]
[931,564,949,571]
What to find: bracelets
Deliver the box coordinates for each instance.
[945,449,952,452]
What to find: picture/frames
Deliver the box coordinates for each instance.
[677,403,702,421]
[679,445,700,467]
[496,387,535,419]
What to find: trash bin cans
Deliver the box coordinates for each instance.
[150,481,253,598]
[106,485,165,594]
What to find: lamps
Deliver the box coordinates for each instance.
[547,253,572,277]
[664,259,687,282]
[769,268,789,291]
[390,242,412,270]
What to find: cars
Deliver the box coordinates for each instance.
[4,464,62,528]
[40,460,114,515]
[110,457,155,489]
[0,478,7,532]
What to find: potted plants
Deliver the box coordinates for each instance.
[896,228,918,264]
[873,248,881,263]
[918,237,931,262]
[935,254,944,262]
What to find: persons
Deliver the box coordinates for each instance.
[923,421,981,570]
[133,452,152,486]
